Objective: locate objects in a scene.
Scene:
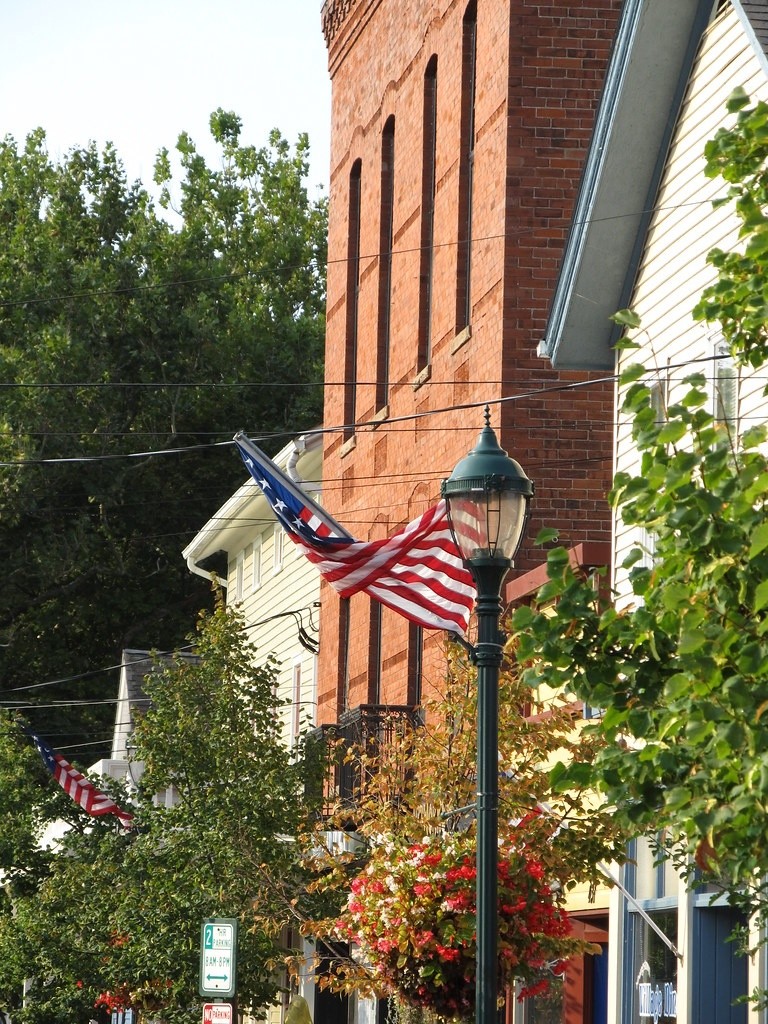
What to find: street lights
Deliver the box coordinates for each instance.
[441,402,534,1024]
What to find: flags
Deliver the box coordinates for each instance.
[232,434,485,638]
[498,757,571,895]
[32,735,133,830]
